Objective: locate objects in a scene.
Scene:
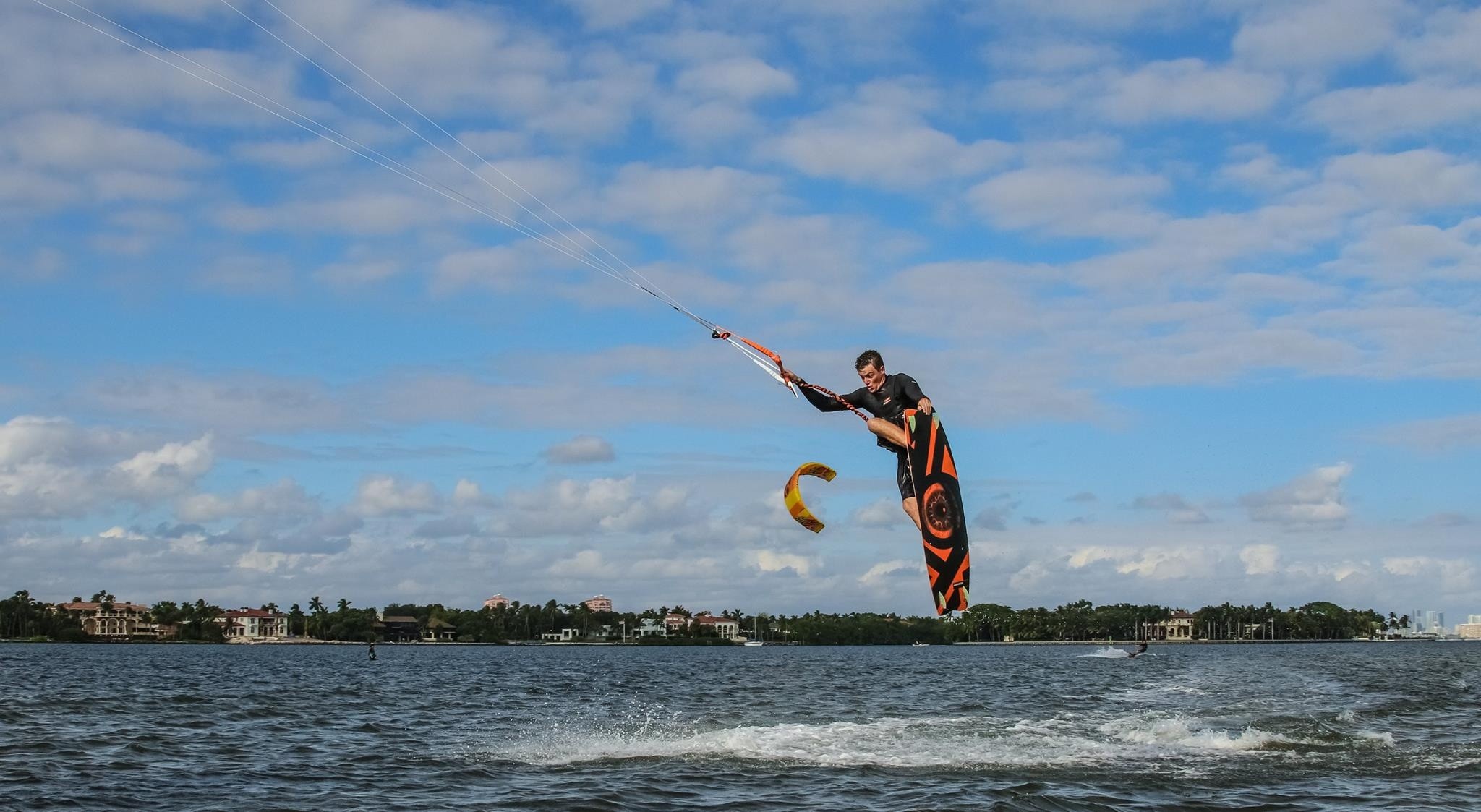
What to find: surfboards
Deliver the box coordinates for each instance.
[895,409,975,619]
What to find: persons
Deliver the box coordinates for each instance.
[368,643,375,657]
[780,350,932,532]
[1131,639,1148,657]
